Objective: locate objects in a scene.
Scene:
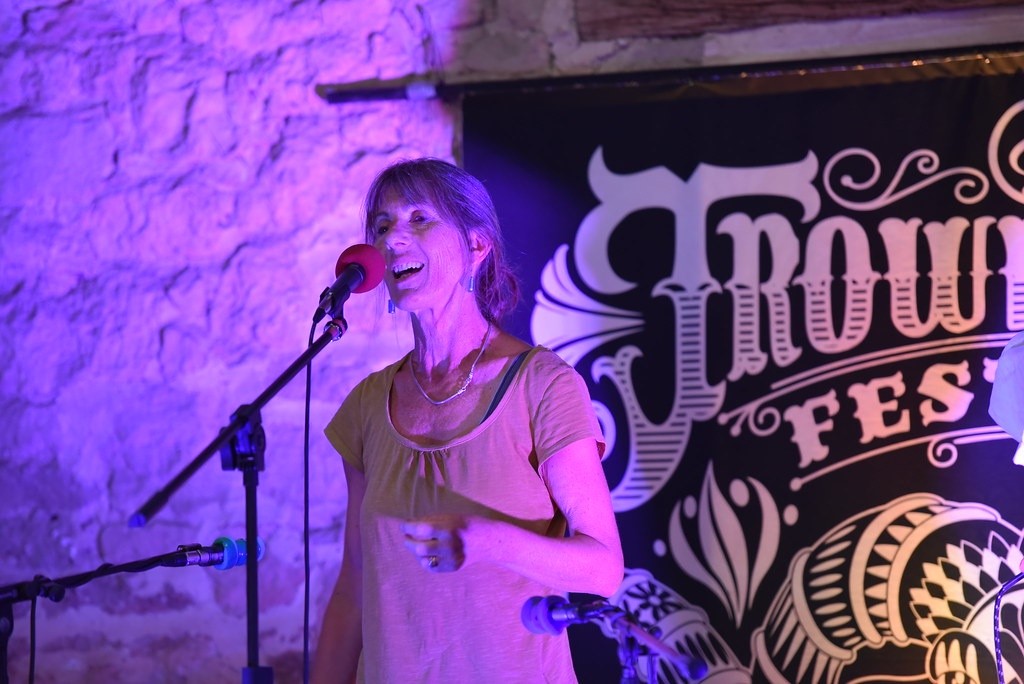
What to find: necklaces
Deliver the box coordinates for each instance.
[410,321,492,404]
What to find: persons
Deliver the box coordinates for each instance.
[308,158,625,684]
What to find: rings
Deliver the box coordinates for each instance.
[429,557,436,567]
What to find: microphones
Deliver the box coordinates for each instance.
[173,535,265,570]
[519,597,598,636]
[313,243,386,324]
